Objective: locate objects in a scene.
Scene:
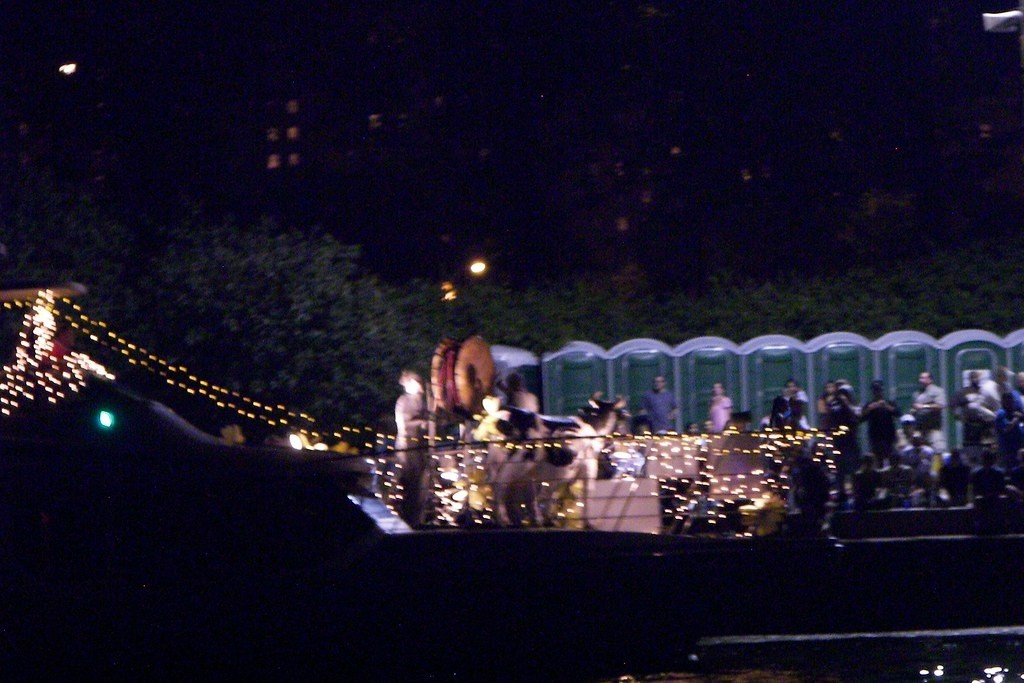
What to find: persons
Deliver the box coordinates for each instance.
[394,368,1024,526]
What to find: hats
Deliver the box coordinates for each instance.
[994,367,1015,376]
[901,415,916,425]
[869,379,885,390]
